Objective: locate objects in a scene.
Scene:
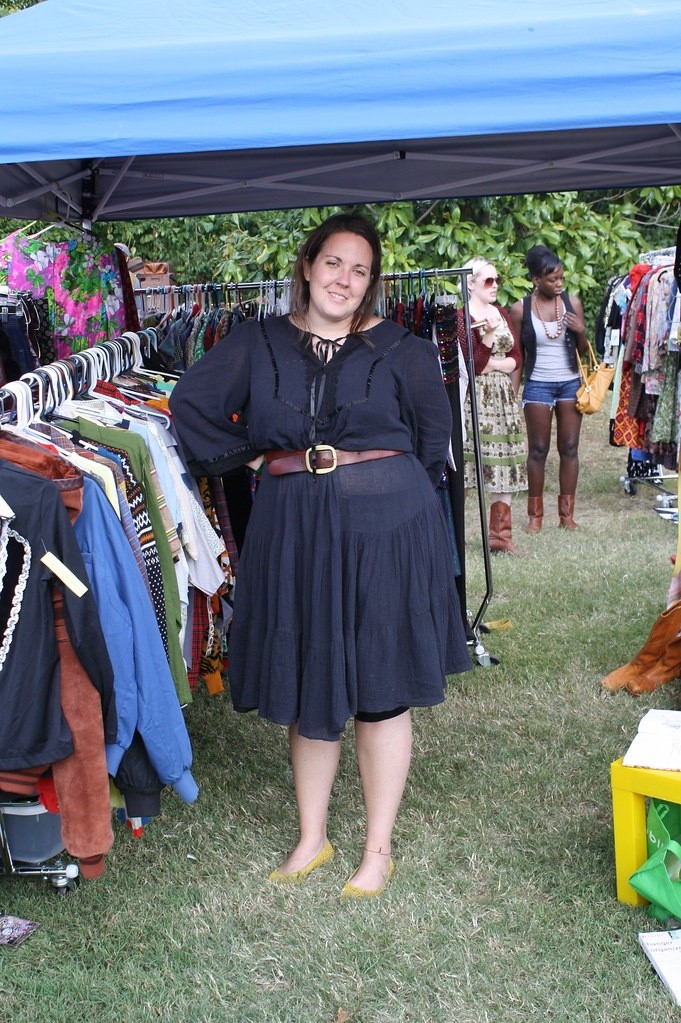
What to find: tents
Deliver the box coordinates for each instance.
[1,1,680,217]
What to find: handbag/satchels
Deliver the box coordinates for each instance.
[575,339,615,415]
[628,797,681,923]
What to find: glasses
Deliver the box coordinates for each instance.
[471,278,501,288]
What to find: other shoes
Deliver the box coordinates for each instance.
[341,857,395,902]
[269,838,334,884]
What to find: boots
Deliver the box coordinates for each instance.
[599,601,681,696]
[526,496,543,536]
[626,633,681,696]
[488,501,511,552]
[558,495,580,529]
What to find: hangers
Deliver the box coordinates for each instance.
[22,179,85,233]
[55,192,84,232]
[25,190,83,238]
[658,268,674,283]
[145,267,448,322]
[0,328,181,457]
[0,290,33,323]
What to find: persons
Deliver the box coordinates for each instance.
[457,255,529,554]
[168,213,474,905]
[510,245,589,534]
[110,243,144,328]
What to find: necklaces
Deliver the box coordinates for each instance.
[533,293,562,339]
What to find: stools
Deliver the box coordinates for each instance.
[609,755,681,907]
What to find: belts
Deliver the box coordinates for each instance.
[266,445,404,475]
